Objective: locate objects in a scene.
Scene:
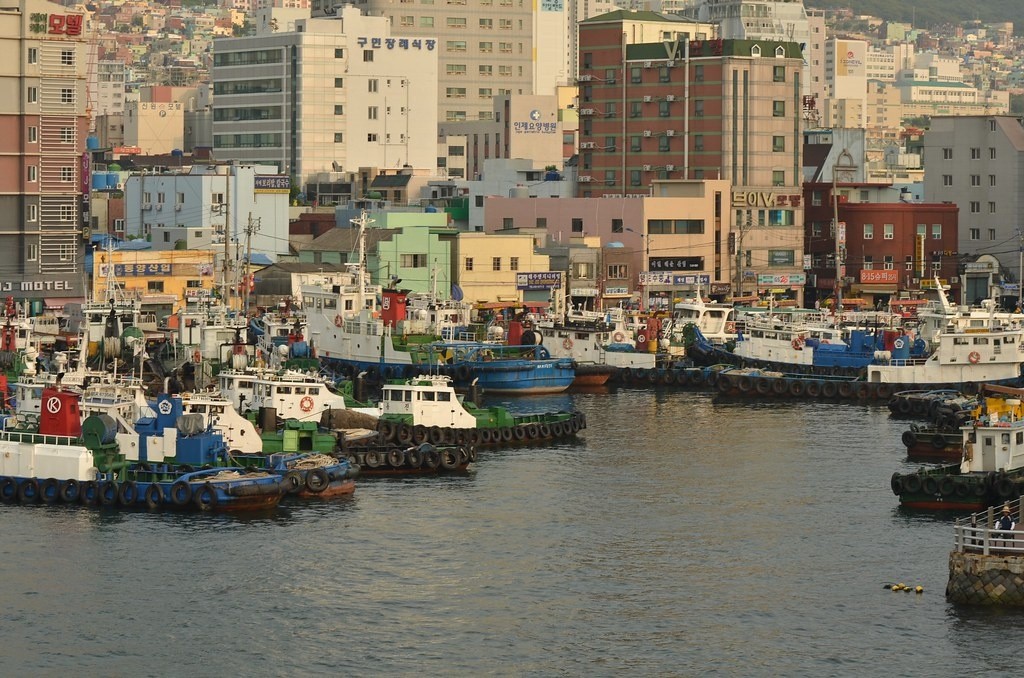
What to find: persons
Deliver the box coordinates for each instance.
[616,299,640,310]
[815,298,820,311]
[767,298,779,310]
[877,300,882,311]
[991,506,1015,539]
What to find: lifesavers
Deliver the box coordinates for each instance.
[194,350,202,364]
[976,421,1012,429]
[906,330,915,341]
[333,314,342,328]
[891,469,1016,498]
[196,485,219,512]
[821,339,829,345]
[300,396,314,412]
[285,470,304,494]
[376,409,588,444]
[145,484,165,509]
[561,337,573,349]
[613,332,624,343]
[968,351,981,365]
[0,475,138,506]
[242,465,277,474]
[886,400,965,448]
[614,366,944,399]
[791,339,803,350]
[306,467,330,492]
[345,443,477,468]
[137,461,214,480]
[570,360,582,370]
[727,320,736,333]
[320,359,472,383]
[171,481,192,506]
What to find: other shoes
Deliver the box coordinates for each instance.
[991,534,994,538]
[1003,534,1008,539]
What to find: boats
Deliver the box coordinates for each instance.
[0,166,1024,514]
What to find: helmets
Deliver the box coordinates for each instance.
[1002,506,1010,512]
